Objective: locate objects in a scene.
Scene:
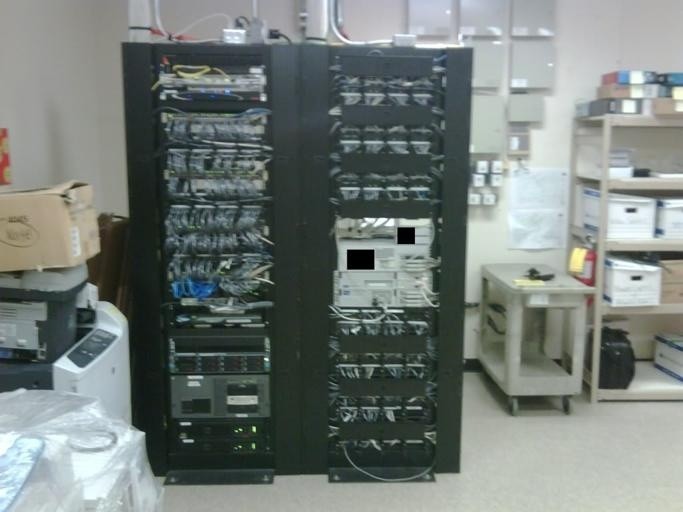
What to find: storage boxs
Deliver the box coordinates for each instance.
[574,182,683,306]
[652,331,683,382]
[0,181,105,272]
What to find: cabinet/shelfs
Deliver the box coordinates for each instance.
[477,264,593,415]
[569,113,683,403]
